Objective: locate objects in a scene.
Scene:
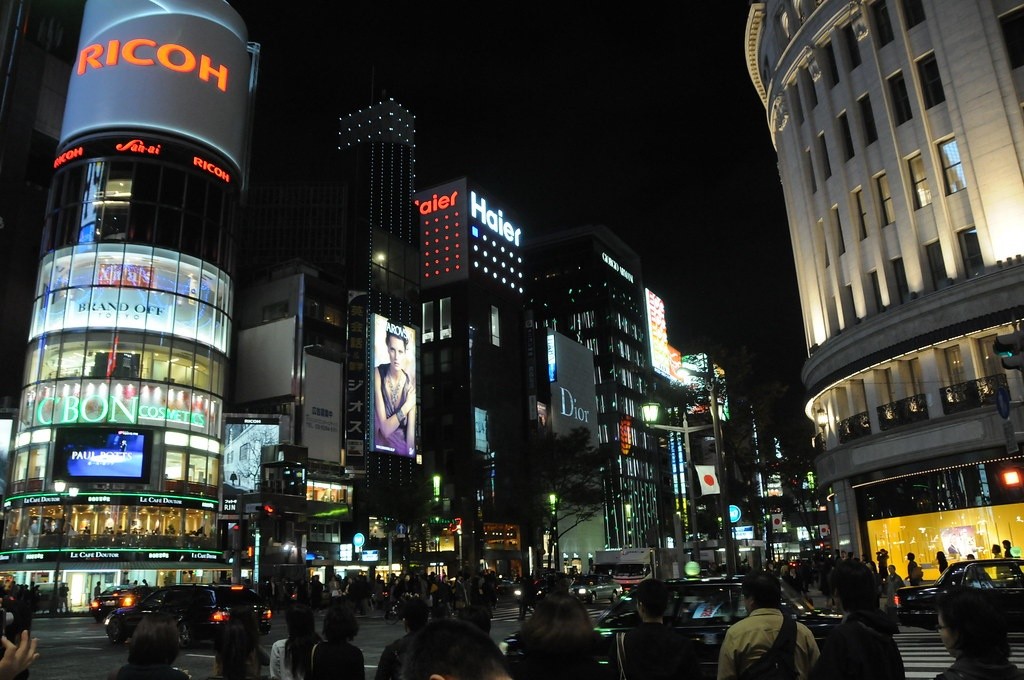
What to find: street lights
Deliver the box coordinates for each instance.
[249,504,273,592]
[638,399,729,572]
[674,367,737,577]
[51,478,79,613]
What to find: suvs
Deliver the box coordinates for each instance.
[104,583,273,649]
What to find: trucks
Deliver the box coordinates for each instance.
[594,547,655,592]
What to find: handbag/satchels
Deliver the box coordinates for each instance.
[741,613,799,680]
[455,600,470,609]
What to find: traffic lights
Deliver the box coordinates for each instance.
[991,330,1024,374]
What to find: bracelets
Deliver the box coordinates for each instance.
[396,409,404,422]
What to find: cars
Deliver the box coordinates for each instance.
[513,572,573,603]
[894,558,1024,628]
[91,585,154,625]
[497,576,844,680]
[569,575,624,606]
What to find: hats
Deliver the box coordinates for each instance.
[627,579,668,614]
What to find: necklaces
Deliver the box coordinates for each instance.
[387,373,402,405]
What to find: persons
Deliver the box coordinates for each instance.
[696,556,753,577]
[103,525,206,537]
[760,549,904,611]
[905,553,923,586]
[513,589,605,680]
[30,517,39,533]
[375,319,416,457]
[936,551,947,573]
[967,554,975,560]
[83,526,90,534]
[0,567,539,680]
[607,579,703,680]
[933,587,1024,680]
[808,559,905,680]
[992,540,1023,579]
[716,571,821,680]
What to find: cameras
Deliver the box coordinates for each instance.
[0,607,14,638]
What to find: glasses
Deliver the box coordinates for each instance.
[935,625,946,633]
